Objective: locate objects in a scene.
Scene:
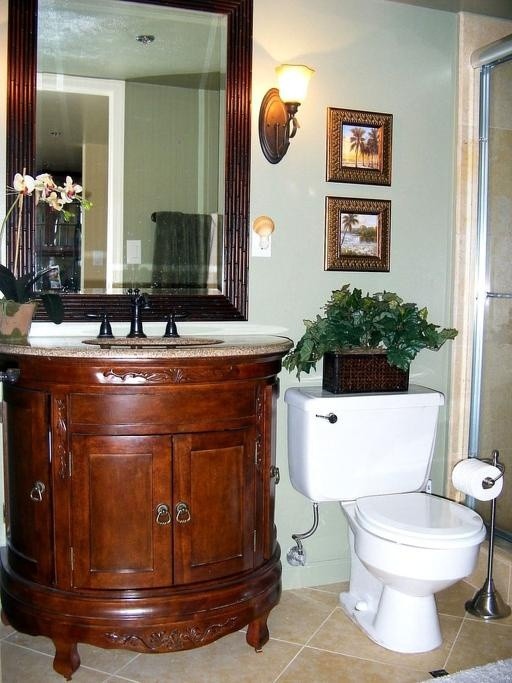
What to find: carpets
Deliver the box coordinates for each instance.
[423,658,512,683]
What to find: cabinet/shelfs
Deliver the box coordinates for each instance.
[0,352,285,680]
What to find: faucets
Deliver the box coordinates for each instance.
[129,293,151,338]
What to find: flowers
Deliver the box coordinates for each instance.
[35,170,93,210]
[0,169,75,326]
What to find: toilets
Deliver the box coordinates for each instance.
[287,383,488,654]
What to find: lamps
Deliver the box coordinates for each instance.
[259,62,316,166]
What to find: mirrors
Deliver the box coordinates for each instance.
[8,2,251,322]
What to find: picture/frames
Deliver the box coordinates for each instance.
[326,107,393,272]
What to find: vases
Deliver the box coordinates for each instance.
[0,297,35,344]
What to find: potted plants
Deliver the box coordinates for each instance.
[282,282,460,397]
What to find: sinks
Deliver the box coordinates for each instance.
[82,338,222,349]
